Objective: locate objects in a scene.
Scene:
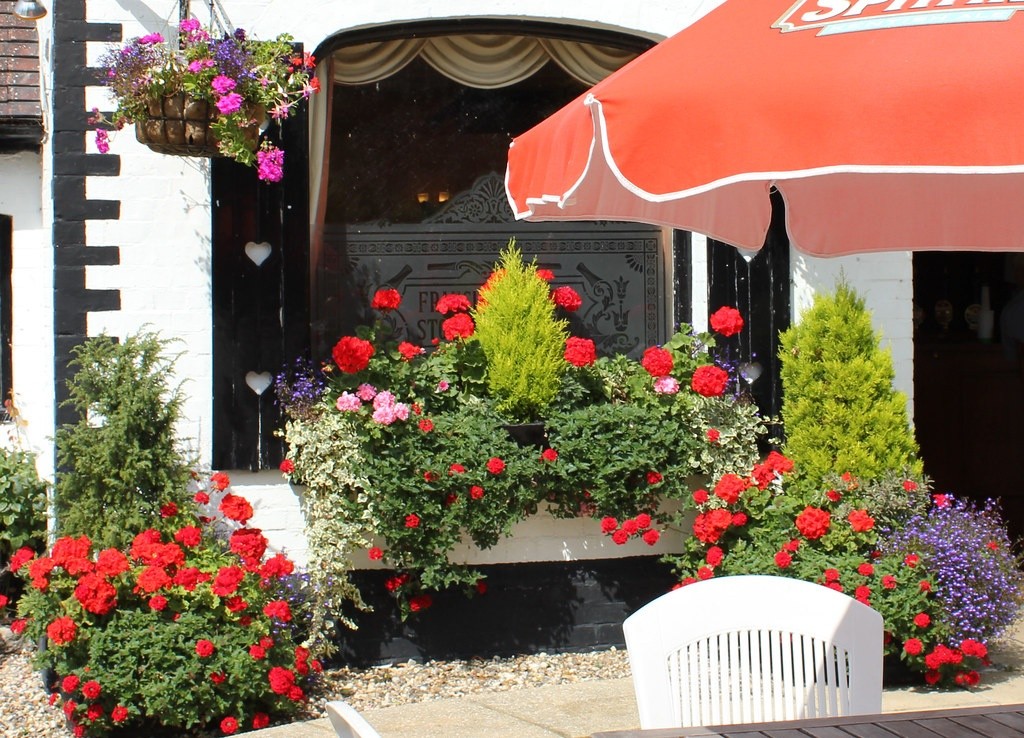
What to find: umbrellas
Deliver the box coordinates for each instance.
[504,0,1024,258]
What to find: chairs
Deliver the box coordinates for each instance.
[623,575,886,730]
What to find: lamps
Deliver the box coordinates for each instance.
[12,0,47,20]
[416,191,449,217]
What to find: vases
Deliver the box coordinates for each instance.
[128,89,265,159]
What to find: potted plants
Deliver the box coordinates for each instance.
[471,232,569,452]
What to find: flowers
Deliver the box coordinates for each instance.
[87,15,321,185]
